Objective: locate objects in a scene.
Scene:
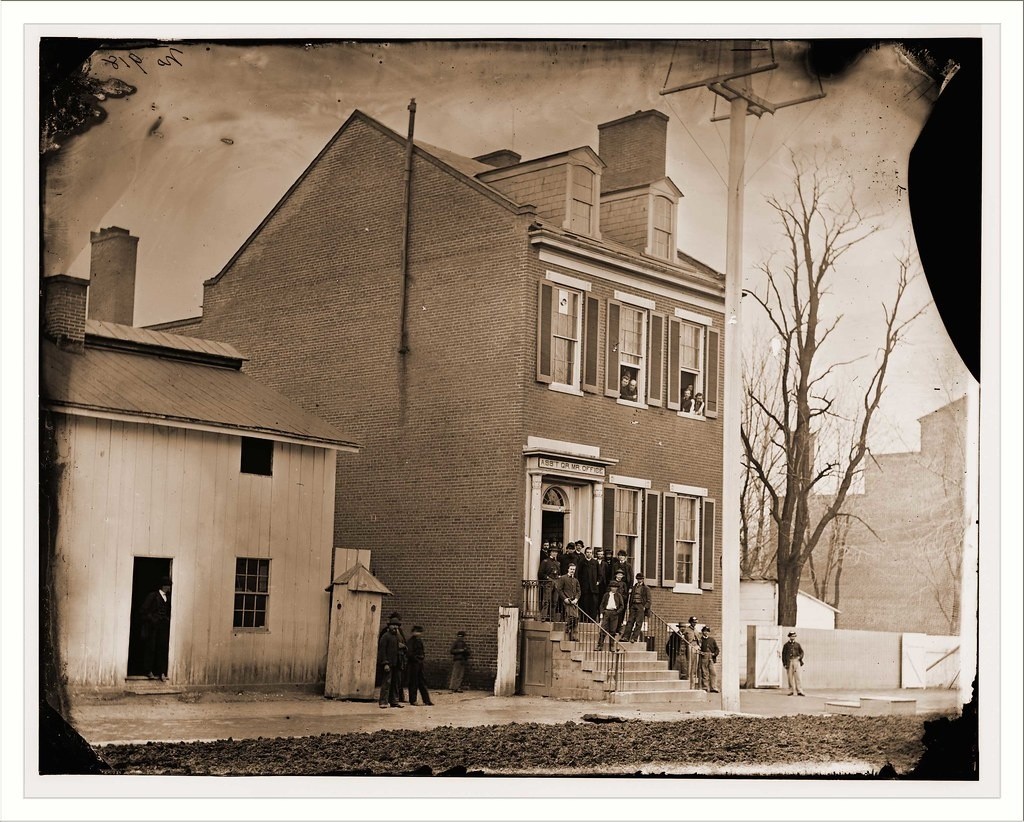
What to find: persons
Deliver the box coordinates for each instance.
[139,575,172,682]
[666,616,719,693]
[681,384,704,416]
[620,369,638,402]
[782,631,806,696]
[379,611,435,708]
[448,631,471,694]
[538,537,652,652]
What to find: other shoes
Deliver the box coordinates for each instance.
[787,692,793,696]
[611,646,615,652]
[595,646,603,651]
[448,689,453,694]
[453,689,464,693]
[630,638,635,643]
[425,701,434,705]
[798,692,805,696]
[410,701,418,705]
[710,690,720,693]
[391,703,400,707]
[619,637,629,641]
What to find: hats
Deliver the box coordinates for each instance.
[548,546,560,551]
[615,568,625,575]
[386,618,402,626]
[576,540,584,548]
[412,626,425,632]
[676,622,688,627]
[688,616,698,623]
[701,626,710,632]
[388,612,402,620]
[636,573,644,579]
[617,550,627,556]
[608,581,620,587]
